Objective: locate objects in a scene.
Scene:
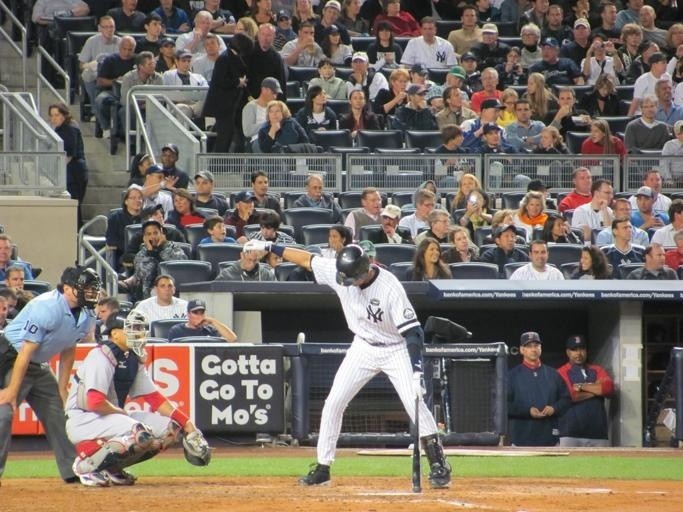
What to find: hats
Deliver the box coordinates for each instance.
[324,0,341,12]
[175,48,192,58]
[352,52,369,62]
[574,18,591,29]
[194,170,214,182]
[462,51,477,61]
[481,23,499,34]
[235,191,257,203]
[158,38,175,47]
[277,10,290,19]
[565,333,588,349]
[411,63,430,74]
[480,98,507,110]
[161,143,179,154]
[327,25,345,33]
[187,299,207,312]
[143,204,162,217]
[448,65,467,80]
[261,76,284,94]
[542,37,559,46]
[495,224,516,236]
[520,331,543,346]
[380,204,402,220]
[483,122,503,134]
[527,180,545,191]
[146,164,172,173]
[634,186,657,197]
[408,85,429,95]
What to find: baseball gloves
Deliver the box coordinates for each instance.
[182,432,209,465]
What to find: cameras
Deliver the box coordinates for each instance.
[597,42,605,48]
[512,62,519,69]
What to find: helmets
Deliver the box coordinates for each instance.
[98,311,150,359]
[61,261,103,309]
[336,244,368,286]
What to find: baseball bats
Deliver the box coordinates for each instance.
[412,371,421,493]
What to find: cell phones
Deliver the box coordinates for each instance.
[469,194,477,203]
[654,214,660,219]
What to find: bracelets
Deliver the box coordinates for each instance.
[410,356,424,372]
[169,407,189,428]
[264,244,286,257]
[161,182,165,189]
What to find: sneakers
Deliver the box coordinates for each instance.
[110,471,138,485]
[72,456,111,486]
[430,472,450,489]
[298,464,330,485]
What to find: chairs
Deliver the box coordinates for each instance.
[0,0,683,341]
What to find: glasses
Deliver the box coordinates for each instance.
[278,17,289,21]
[100,24,114,28]
[598,191,613,194]
[126,196,143,200]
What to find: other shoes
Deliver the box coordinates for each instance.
[102,129,111,139]
[90,116,96,122]
[118,280,130,291]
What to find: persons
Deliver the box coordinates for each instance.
[555,335,614,448]
[133,275,191,329]
[167,299,238,343]
[64,309,211,489]
[95,297,121,341]
[0,235,42,329]
[78,1,683,299]
[31,1,90,42]
[48,103,88,233]
[243,239,452,488]
[506,332,573,449]
[0,265,101,487]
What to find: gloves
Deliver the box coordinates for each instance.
[411,371,427,401]
[243,239,273,253]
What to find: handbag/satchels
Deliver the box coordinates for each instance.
[82,67,98,83]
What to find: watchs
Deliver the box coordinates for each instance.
[577,383,583,392]
